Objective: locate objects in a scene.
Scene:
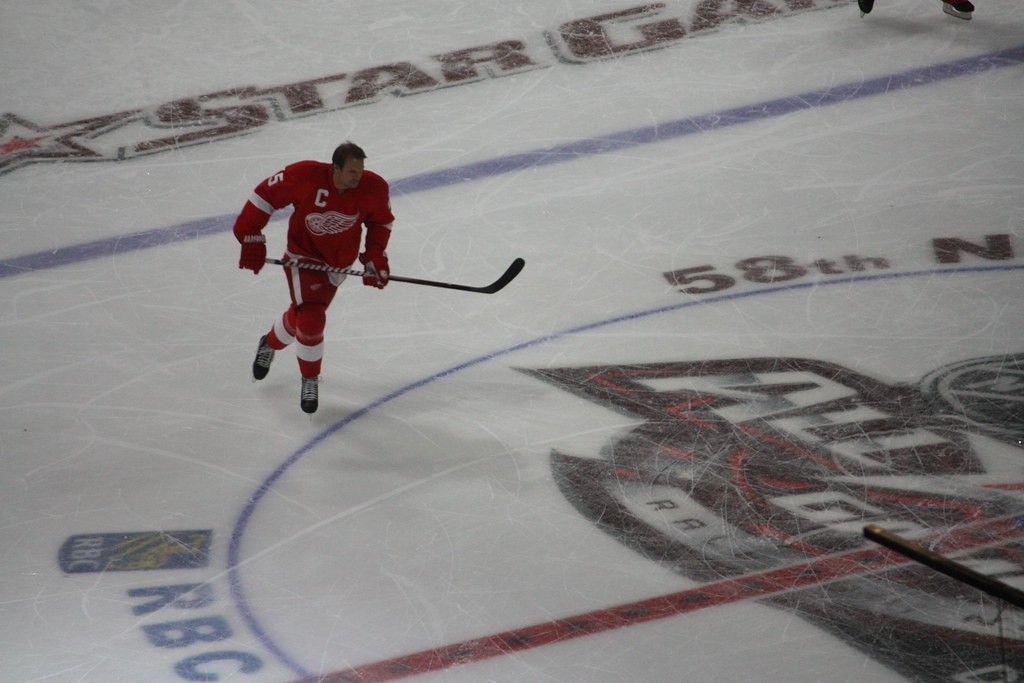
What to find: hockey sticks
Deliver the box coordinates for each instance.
[266,256,525,294]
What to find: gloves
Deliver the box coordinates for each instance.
[358,249,390,289]
[238,231,268,275]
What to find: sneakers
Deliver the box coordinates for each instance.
[300,376,319,422]
[251,335,275,383]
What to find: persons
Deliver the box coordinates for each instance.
[232,139,396,413]
